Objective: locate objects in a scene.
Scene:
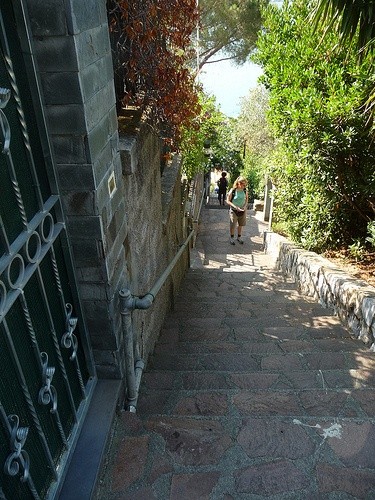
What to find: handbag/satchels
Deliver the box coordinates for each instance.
[232,209,244,217]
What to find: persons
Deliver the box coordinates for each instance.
[218,172,228,205]
[227,176,248,246]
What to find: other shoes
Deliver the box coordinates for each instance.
[237,237,244,243]
[230,237,236,244]
